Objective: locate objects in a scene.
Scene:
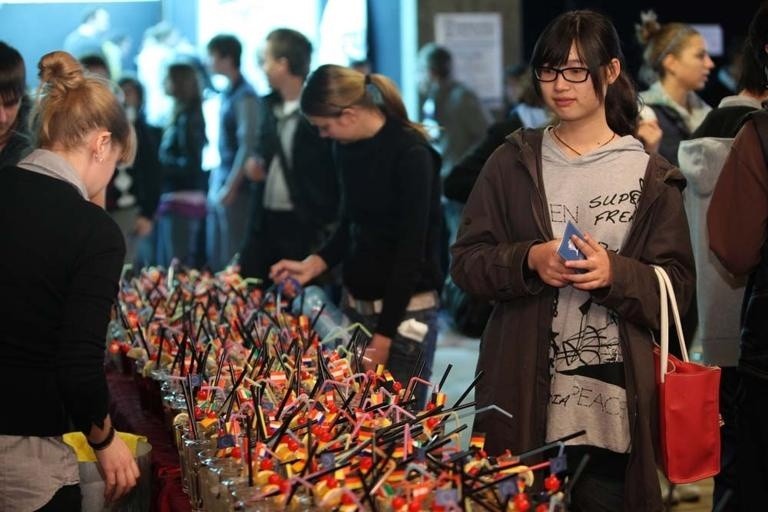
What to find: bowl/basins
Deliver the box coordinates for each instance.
[551,123,617,160]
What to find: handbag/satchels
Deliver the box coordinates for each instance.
[86,423,117,450]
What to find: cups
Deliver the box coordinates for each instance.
[533,65,591,85]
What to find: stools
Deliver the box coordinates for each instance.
[341,292,441,316]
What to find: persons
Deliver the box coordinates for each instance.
[442,68,556,207]
[706,1,767,511]
[0,40,41,167]
[266,63,451,412]
[676,42,753,511]
[449,8,699,512]
[1,50,142,512]
[104,76,163,239]
[633,9,716,167]
[242,27,340,317]
[418,42,489,243]
[154,62,208,274]
[504,60,532,108]
[75,55,113,84]
[204,33,265,278]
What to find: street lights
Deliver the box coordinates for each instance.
[650,265,722,484]
